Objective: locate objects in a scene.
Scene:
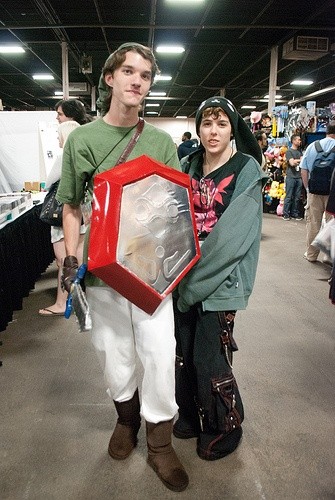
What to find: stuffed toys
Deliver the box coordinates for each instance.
[264,145,288,216]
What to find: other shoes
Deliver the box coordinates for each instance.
[283,215,290,220]
[304,251,317,261]
[172,419,199,439]
[197,424,243,461]
[290,215,302,220]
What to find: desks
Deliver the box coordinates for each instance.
[0,190,56,333]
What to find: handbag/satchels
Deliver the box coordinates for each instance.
[39,173,90,227]
[311,215,335,263]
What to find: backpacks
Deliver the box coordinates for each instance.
[308,139,335,195]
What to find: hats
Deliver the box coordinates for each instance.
[195,96,263,172]
[261,115,271,121]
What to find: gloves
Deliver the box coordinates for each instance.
[59,255,79,292]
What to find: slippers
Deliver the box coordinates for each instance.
[38,308,65,316]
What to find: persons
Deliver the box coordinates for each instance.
[55,42,189,493]
[255,131,268,173]
[310,168,335,304]
[300,124,335,261]
[283,135,303,221]
[172,95,273,462]
[177,132,199,160]
[39,98,96,318]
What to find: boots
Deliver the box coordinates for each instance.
[144,420,190,492]
[107,387,142,460]
